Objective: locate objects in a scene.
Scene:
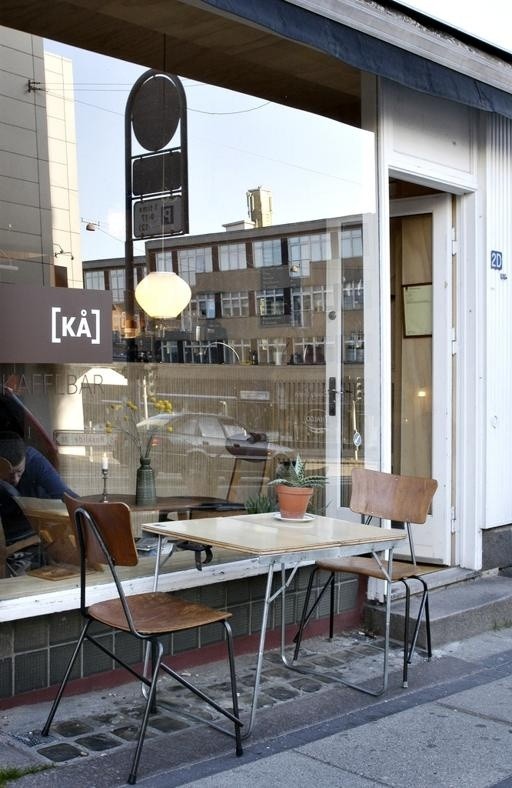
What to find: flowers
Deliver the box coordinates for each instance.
[105,398,175,457]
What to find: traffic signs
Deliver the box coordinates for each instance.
[55,430,117,446]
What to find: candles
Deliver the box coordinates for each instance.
[101,452,109,502]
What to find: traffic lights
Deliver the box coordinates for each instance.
[355,376,362,402]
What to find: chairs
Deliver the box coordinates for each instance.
[41,490,242,784]
[228,459,266,507]
[293,468,438,688]
[0,519,43,579]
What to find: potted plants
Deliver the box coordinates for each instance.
[267,453,329,519]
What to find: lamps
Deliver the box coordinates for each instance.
[129,68,191,320]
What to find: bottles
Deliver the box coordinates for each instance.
[251,331,365,365]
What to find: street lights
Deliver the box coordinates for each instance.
[199,342,242,424]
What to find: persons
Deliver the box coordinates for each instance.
[0,430,80,500]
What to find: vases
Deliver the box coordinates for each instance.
[135,458,156,507]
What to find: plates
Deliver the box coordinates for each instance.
[272,512,317,523]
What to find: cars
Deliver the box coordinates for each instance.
[114,412,304,488]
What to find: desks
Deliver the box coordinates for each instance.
[77,494,228,548]
[141,511,407,737]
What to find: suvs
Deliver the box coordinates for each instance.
[0,386,59,479]
